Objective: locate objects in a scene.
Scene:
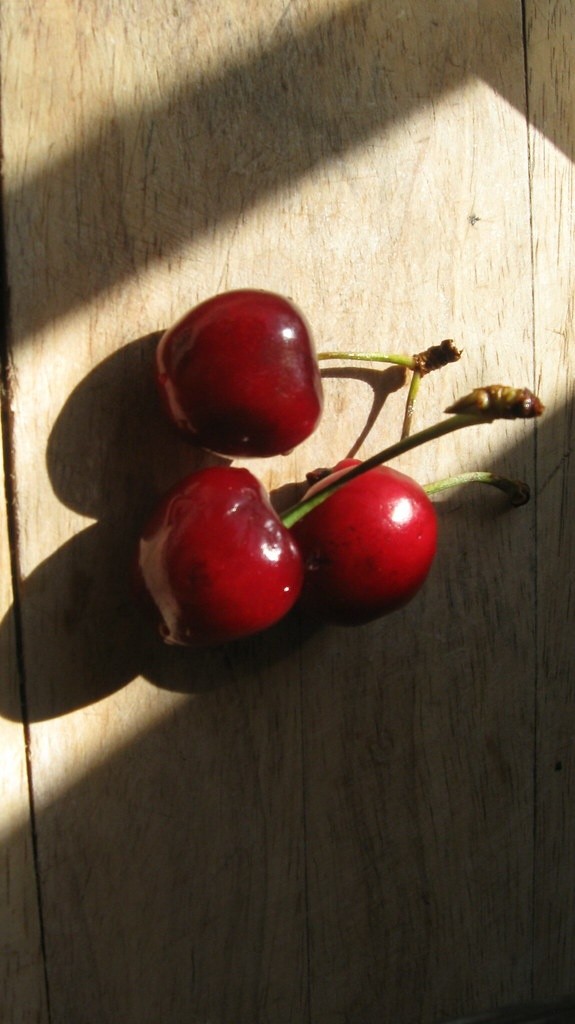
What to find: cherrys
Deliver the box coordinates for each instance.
[136,290,543,643]
